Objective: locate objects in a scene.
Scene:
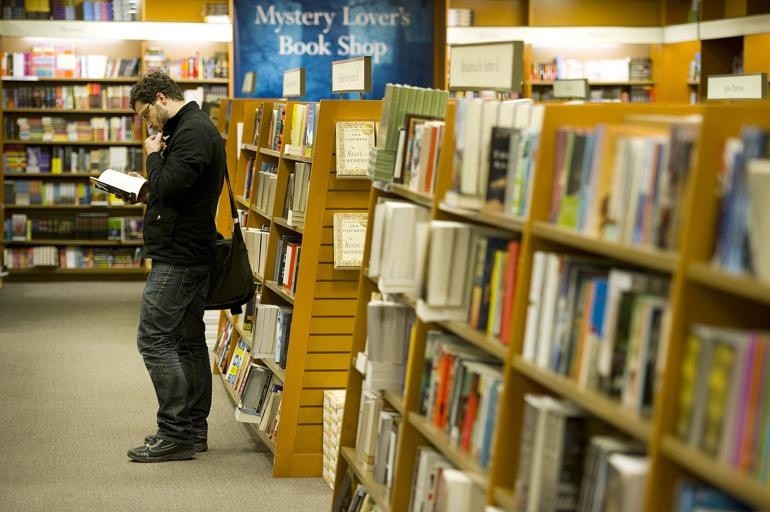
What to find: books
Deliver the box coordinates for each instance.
[211,100,321,442]
[0,0,142,21]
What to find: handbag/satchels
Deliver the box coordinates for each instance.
[204,223,255,314]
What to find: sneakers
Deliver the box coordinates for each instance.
[128,434,207,461]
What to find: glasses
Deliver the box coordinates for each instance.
[140,92,158,121]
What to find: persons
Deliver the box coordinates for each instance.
[112,71,228,461]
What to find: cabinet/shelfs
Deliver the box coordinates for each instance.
[0,36,231,281]
[212,36,770,512]
